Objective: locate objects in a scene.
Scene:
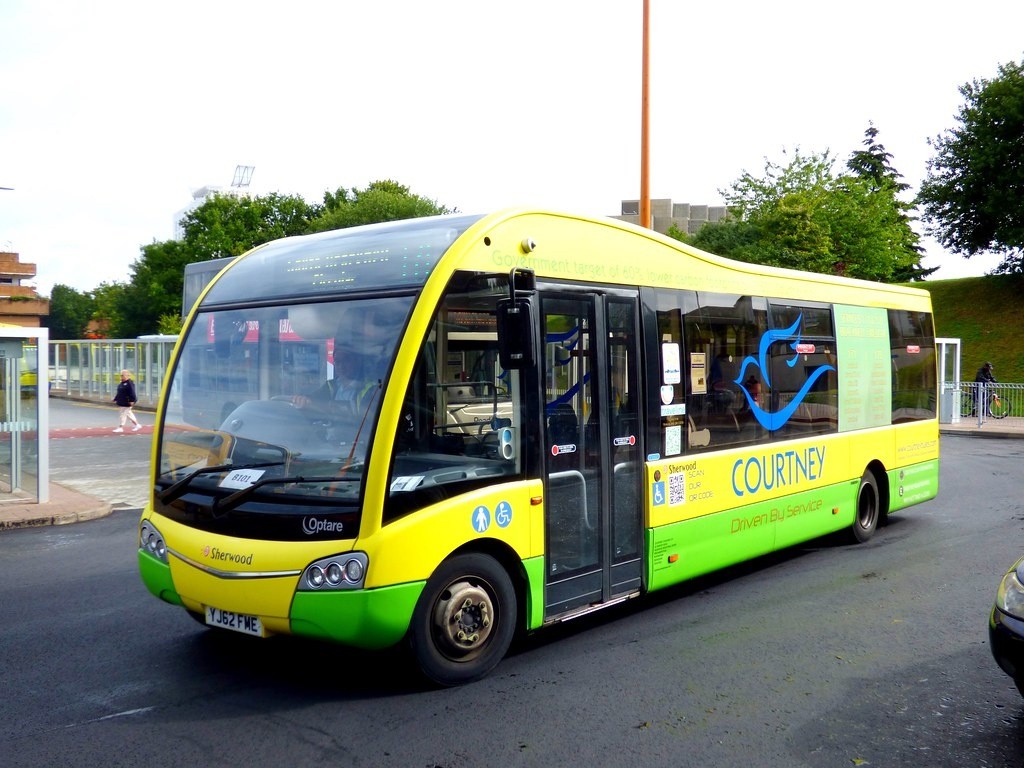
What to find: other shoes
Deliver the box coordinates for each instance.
[972,414,978,417]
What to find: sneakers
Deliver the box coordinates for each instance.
[112,428,123,432]
[132,424,143,431]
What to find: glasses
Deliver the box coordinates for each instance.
[333,357,353,368]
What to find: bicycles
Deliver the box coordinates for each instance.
[960,382,1012,419]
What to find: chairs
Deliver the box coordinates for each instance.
[686,393,811,451]
[548,403,579,452]
[584,404,620,454]
[332,305,428,443]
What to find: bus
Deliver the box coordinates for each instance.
[182,254,565,434]
[138,208,941,686]
[0,345,52,398]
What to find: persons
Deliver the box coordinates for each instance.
[112,370,142,433]
[972,361,999,416]
[292,343,381,450]
[739,375,763,414]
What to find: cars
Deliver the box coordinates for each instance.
[989,555,1024,695]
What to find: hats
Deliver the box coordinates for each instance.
[985,362,993,370]
